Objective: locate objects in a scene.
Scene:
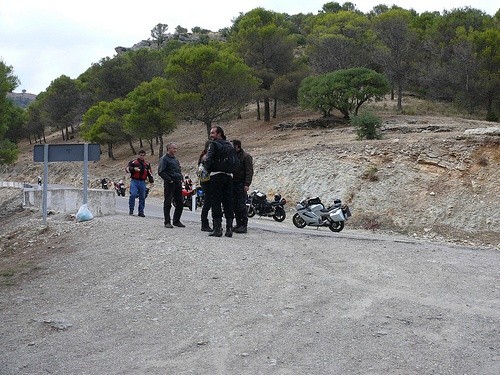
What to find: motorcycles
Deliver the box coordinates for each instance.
[130,185,150,199]
[193,186,204,209]
[246,190,286,223]
[292,196,352,232]
[172,183,196,211]
[114,181,126,197]
[100,178,108,190]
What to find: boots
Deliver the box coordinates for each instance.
[201,219,214,232]
[225,218,233,236]
[209,217,223,237]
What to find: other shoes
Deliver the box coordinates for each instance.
[173,220,185,227]
[165,223,173,228]
[234,224,247,233]
[130,209,133,214]
[138,213,145,217]
[232,224,240,232]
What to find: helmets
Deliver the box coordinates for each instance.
[275,193,281,201]
[333,199,342,208]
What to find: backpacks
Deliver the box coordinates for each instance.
[212,141,242,174]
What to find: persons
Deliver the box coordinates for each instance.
[116,180,125,192]
[101,178,107,188]
[37,177,42,186]
[196,126,253,237]
[125,150,155,217]
[184,175,192,190]
[157,143,185,228]
